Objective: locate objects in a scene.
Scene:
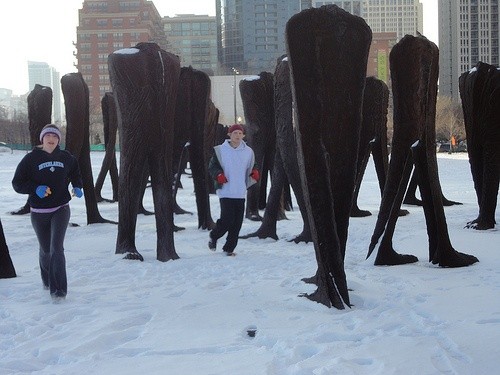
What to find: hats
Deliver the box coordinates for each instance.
[40,124,61,142]
[229,124,243,132]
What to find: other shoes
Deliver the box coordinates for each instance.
[222,249,236,257]
[208,232,217,251]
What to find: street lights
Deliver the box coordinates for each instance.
[232,67,240,124]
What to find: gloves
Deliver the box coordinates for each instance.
[249,168,260,181]
[72,188,83,198]
[36,185,51,198]
[217,173,228,185]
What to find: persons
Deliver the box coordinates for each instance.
[11,124,82,304]
[208,125,259,257]
